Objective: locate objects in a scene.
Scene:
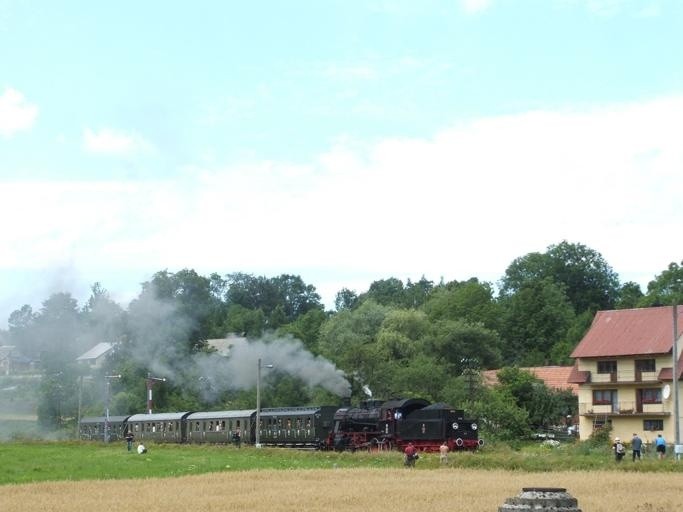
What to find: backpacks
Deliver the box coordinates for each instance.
[616,442,626,455]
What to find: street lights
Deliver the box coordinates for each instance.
[146,372,166,414]
[104,375,121,443]
[255,358,273,448]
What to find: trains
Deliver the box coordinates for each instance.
[77,397,485,453]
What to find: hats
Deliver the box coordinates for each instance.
[615,437,620,441]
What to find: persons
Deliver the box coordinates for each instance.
[439,441,449,465]
[234,428,242,449]
[138,442,147,455]
[125,430,134,452]
[631,433,642,462]
[655,434,666,460]
[612,437,625,462]
[386,409,402,421]
[404,443,419,468]
